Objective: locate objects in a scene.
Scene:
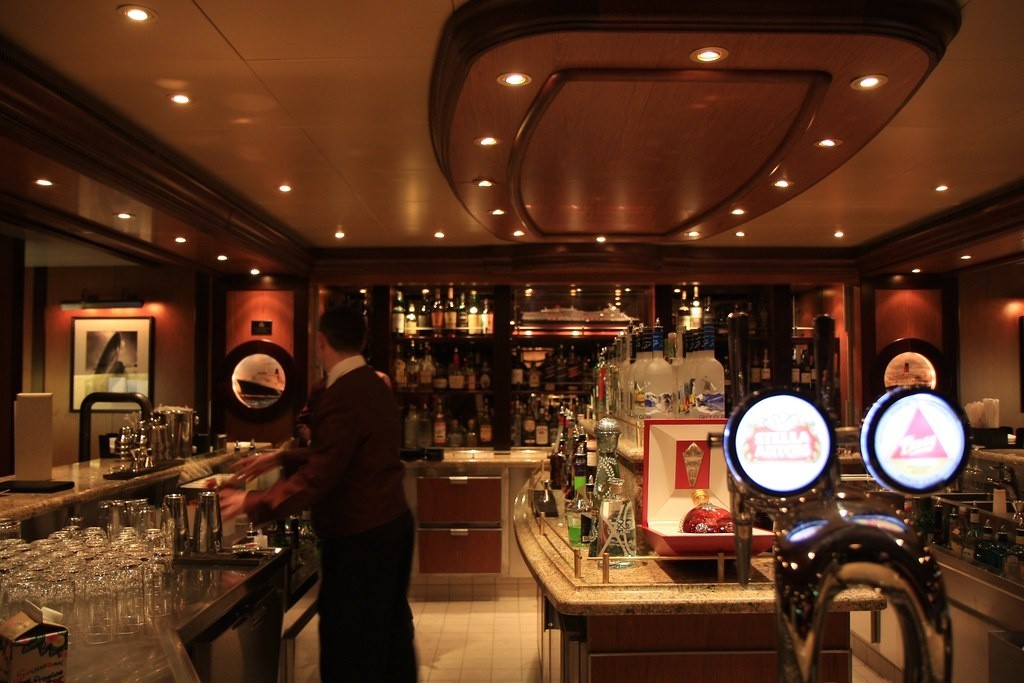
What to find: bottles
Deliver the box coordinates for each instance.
[838,445,865,474]
[781,585,819,642]
[405,395,493,447]
[759,304,769,336]
[512,286,744,570]
[394,287,493,335]
[245,509,316,573]
[762,348,771,388]
[899,498,1024,580]
[233,440,243,469]
[746,302,756,336]
[398,340,491,389]
[751,348,761,391]
[791,348,817,387]
[247,439,258,492]
[192,416,201,455]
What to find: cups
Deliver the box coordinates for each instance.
[112,402,192,471]
[0,493,189,644]
[195,492,222,552]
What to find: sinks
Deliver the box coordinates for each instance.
[933,493,1000,501]
[961,502,1023,513]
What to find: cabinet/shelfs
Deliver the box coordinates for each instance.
[414,470,504,576]
[849,575,1007,683]
[536,578,849,683]
[361,284,653,450]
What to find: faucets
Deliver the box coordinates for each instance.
[984,466,1021,502]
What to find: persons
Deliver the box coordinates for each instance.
[219,302,418,683]
[293,365,392,448]
[87,331,128,373]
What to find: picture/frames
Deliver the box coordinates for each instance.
[878,338,944,401]
[71,315,155,412]
[218,339,298,423]
